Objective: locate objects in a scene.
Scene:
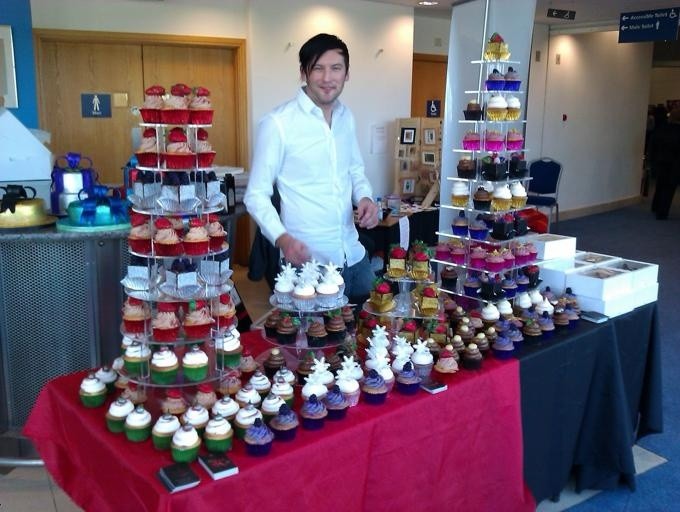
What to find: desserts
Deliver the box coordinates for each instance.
[77,32,580,465]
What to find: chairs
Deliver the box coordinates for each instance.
[523,158,565,228]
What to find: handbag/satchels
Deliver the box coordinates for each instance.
[640,170,649,197]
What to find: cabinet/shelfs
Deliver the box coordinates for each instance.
[113,122,244,408]
[360,273,443,320]
[261,293,354,363]
[427,57,551,305]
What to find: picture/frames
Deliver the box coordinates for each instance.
[0,23,19,110]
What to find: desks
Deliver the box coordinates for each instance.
[20,300,664,512]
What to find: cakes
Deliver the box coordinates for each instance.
[50,167,128,226]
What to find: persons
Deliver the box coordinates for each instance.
[243,34,380,303]
